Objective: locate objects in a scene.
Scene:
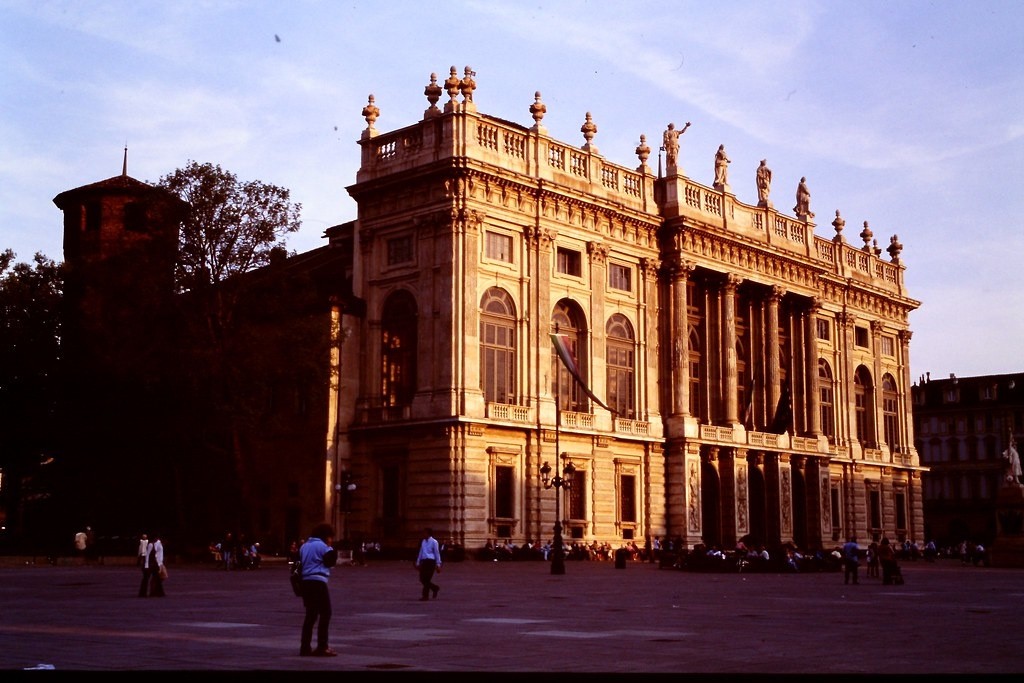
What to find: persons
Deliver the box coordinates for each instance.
[439,536,990,585]
[295,524,353,657]
[136,530,306,602]
[74,521,97,566]
[360,538,381,561]
[416,527,442,601]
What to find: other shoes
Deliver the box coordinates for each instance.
[433,586,439,598]
[300,648,337,657]
